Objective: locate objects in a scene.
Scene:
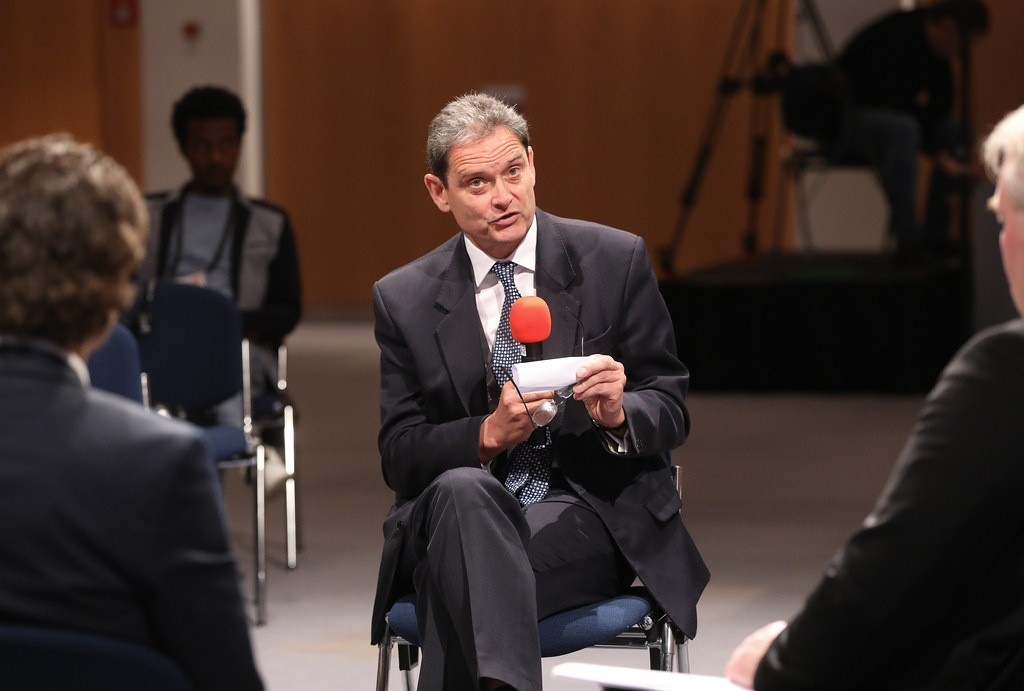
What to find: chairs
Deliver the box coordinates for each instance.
[377,464,684,691]
[86,324,299,626]
[775,65,901,269]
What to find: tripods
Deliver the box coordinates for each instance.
[658,1,836,276]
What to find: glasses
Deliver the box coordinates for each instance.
[501,306,582,429]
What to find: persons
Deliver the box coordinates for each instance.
[370,91,712,691]
[130,85,303,499]
[830,0,992,251]
[730,101,1024,691]
[0,134,265,691]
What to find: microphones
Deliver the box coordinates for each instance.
[511,297,552,447]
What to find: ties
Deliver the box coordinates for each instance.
[488,261,554,508]
